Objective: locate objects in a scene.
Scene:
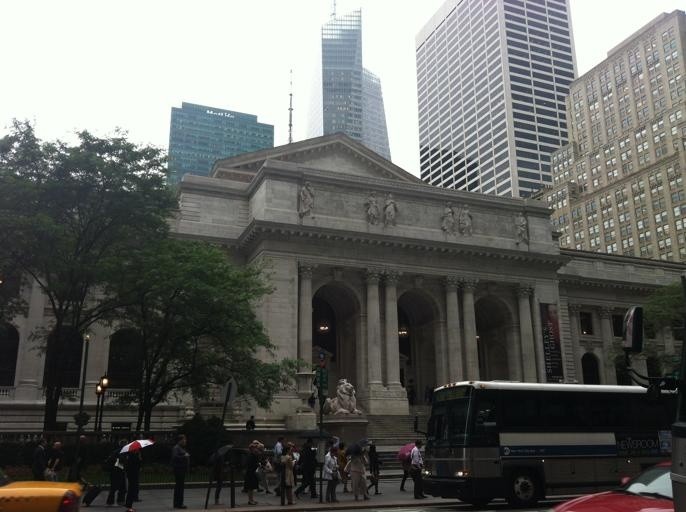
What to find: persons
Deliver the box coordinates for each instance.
[382,193,400,229]
[364,191,382,228]
[401,379,438,406]
[242,436,382,505]
[441,202,459,238]
[400,440,427,499]
[31,438,101,505]
[308,383,320,408]
[172,437,188,509]
[246,415,257,432]
[214,454,229,503]
[106,440,142,510]
[458,203,475,239]
[546,305,560,347]
[514,212,530,247]
[300,181,316,221]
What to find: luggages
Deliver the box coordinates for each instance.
[82,486,100,505]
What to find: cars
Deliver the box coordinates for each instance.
[0,466,84,512]
[545,454,676,512]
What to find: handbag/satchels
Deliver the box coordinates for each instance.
[262,461,273,473]
[115,458,124,470]
[344,460,351,473]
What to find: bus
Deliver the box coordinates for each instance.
[413,377,685,507]
[621,267,686,512]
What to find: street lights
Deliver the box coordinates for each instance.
[308,368,332,505]
[67,326,114,483]
